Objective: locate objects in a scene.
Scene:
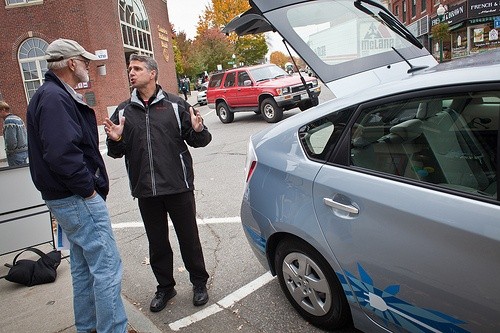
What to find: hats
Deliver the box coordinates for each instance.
[45,38,101,62]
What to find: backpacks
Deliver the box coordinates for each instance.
[4,248,62,287]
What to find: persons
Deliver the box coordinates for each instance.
[194,79,202,90]
[180,79,189,101]
[202,75,205,84]
[204,75,210,82]
[103,54,213,313]
[0,100,29,167]
[25,37,139,333]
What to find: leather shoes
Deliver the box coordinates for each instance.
[193,286,209,306]
[150,287,177,312]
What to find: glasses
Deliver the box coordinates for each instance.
[76,59,89,66]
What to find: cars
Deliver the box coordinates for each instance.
[221,0,500,332]
[194,79,202,90]
[197,84,207,106]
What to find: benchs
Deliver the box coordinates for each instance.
[351,101,495,195]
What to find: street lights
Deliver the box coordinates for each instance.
[436,4,446,63]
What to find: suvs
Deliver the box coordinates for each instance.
[206,64,321,123]
